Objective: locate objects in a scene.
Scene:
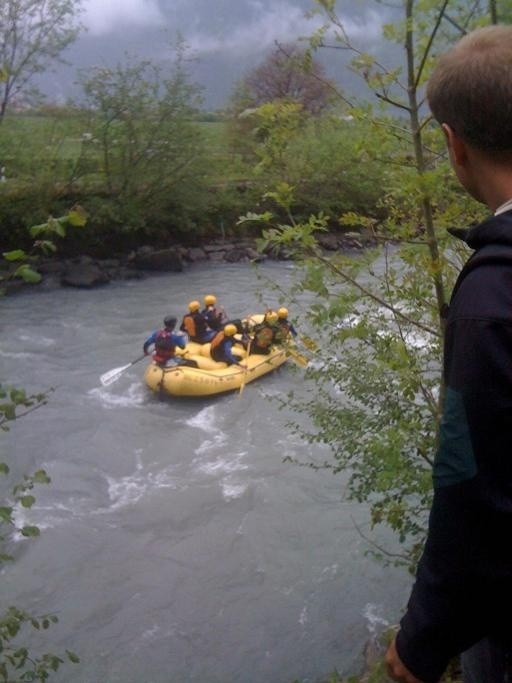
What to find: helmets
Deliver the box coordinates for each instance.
[188,300,200,313]
[223,323,238,337]
[203,294,216,306]
[278,307,289,320]
[162,315,178,327]
[264,310,278,326]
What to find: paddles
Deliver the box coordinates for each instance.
[239,339,252,393]
[248,317,308,368]
[99,347,155,387]
[294,332,318,353]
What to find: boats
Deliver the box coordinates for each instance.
[143,314,291,396]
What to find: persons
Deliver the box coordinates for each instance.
[144,316,200,369]
[382,24,512,683]
[179,294,297,368]
